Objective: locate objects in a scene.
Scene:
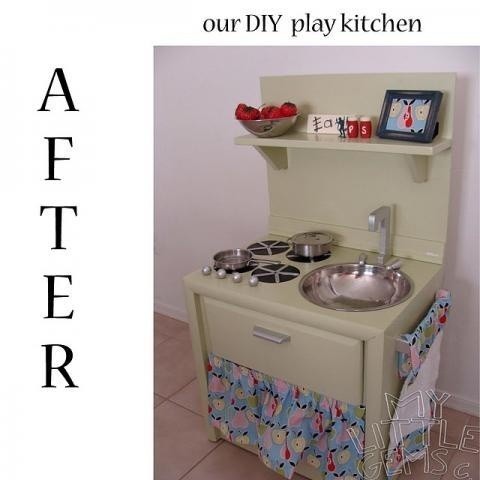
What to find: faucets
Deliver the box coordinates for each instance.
[367,205,391,265]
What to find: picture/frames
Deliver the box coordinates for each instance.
[375,89,442,143]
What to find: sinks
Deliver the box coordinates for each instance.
[297,253,414,313]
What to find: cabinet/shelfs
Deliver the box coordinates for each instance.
[185,267,446,480]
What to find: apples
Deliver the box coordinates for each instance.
[235,102,296,120]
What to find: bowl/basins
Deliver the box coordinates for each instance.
[234,112,301,137]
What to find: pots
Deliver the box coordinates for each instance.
[213,248,280,271]
[287,231,336,257]
